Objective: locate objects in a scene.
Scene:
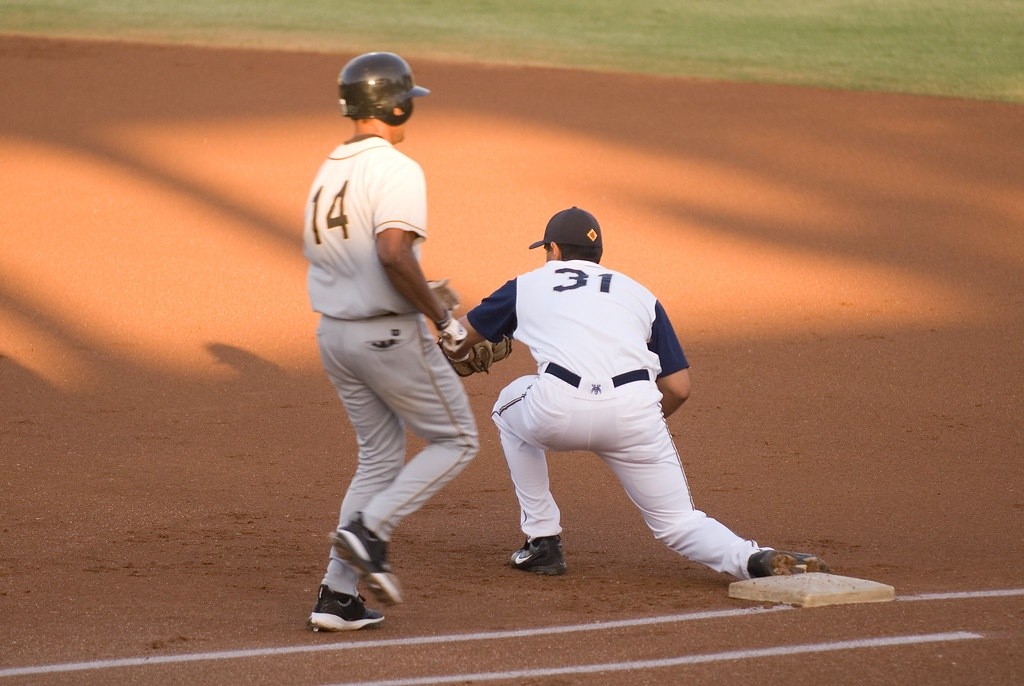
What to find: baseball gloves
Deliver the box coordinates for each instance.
[436,326,511,378]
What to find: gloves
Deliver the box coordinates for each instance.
[427,277,460,313]
[436,309,469,352]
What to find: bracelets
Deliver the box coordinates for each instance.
[448,354,469,362]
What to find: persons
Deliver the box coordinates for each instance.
[449,206,829,580]
[304,51,479,632]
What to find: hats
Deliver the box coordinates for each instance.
[529,207,603,249]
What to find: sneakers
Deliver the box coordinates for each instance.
[511,535,567,574]
[307,584,385,632]
[329,510,403,604]
[748,549,834,579]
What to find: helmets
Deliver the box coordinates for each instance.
[338,51,431,127]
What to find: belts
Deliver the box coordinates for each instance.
[545,362,650,388]
